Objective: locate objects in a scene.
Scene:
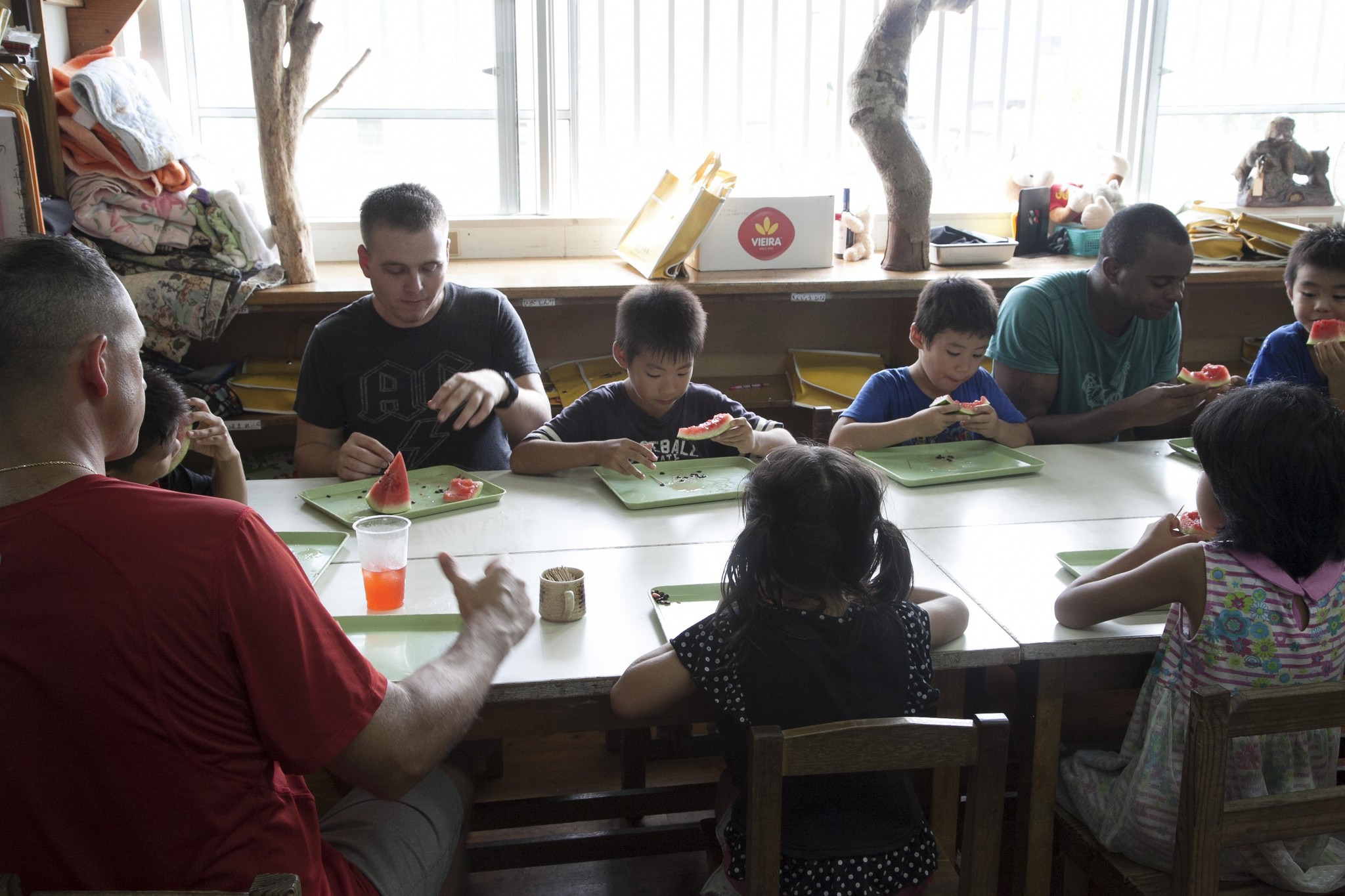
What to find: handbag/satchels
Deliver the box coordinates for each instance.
[1175,200,1317,261]
[614,150,738,280]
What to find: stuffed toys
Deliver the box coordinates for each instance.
[1000,153,1128,231]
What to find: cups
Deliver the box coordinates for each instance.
[352,515,412,612]
[539,567,587,623]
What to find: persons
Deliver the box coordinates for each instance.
[289,184,553,478]
[985,203,1247,444]
[1247,224,1345,416]
[602,445,969,896]
[828,275,1034,455]
[1056,378,1344,896]
[0,234,539,896]
[104,365,247,507]
[509,282,800,483]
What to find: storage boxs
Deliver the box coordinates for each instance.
[928,230,1019,266]
[1053,225,1103,256]
[684,195,834,272]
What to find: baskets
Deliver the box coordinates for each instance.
[1053,226,1102,256]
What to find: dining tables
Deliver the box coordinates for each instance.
[245,461,1022,896]
[882,436,1203,896]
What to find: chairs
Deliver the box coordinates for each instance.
[698,712,1010,896]
[1052,680,1345,896]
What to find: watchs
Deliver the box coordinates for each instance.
[496,370,518,409]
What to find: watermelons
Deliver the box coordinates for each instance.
[1306,318,1345,345]
[365,450,412,513]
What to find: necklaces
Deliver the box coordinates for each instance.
[0,461,97,474]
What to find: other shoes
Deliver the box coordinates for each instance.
[450,740,505,778]
